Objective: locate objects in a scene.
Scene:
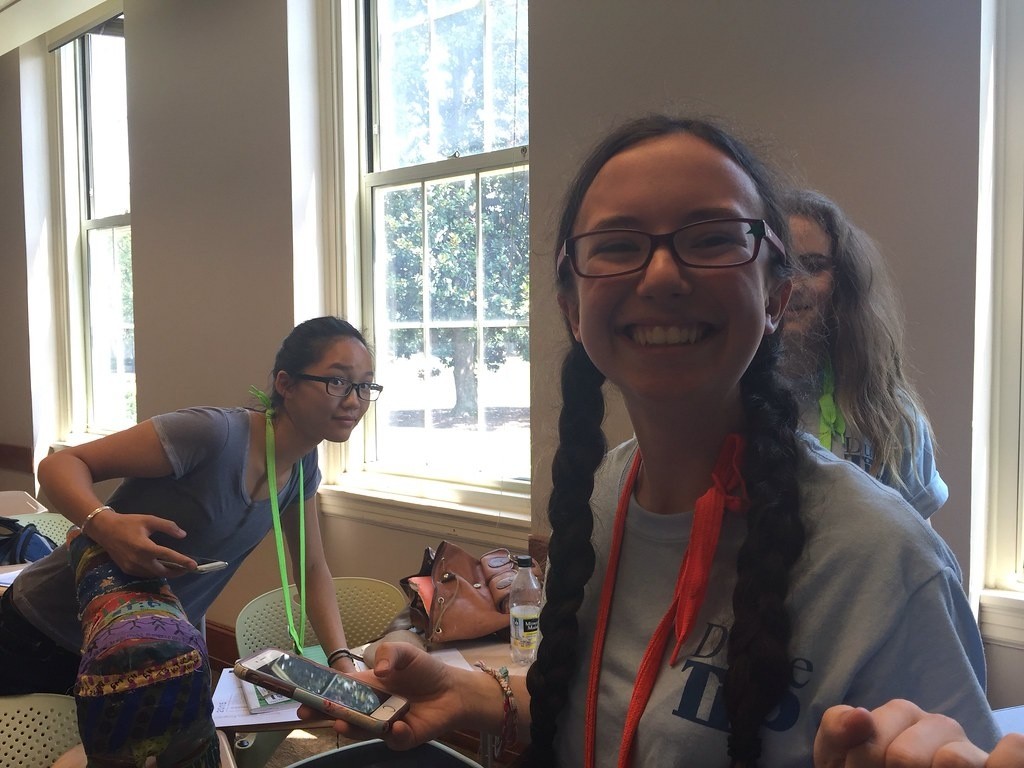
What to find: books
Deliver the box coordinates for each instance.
[232,644,329,714]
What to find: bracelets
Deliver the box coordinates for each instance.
[474,659,520,748]
[79,505,114,535]
[327,647,354,669]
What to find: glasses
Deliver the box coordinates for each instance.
[289,372,385,402]
[556,218,787,284]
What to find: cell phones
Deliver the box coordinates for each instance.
[233,646,411,733]
[158,555,230,575]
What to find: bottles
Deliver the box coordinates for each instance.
[509,554,542,665]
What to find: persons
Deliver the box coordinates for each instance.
[293,111,1024,766]
[2,317,358,697]
[767,186,951,528]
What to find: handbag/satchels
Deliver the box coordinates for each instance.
[0,516,59,565]
[399,539,545,643]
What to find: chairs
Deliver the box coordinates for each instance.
[235,576,410,659]
[0,692,80,767]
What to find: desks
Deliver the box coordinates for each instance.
[348,627,534,675]
